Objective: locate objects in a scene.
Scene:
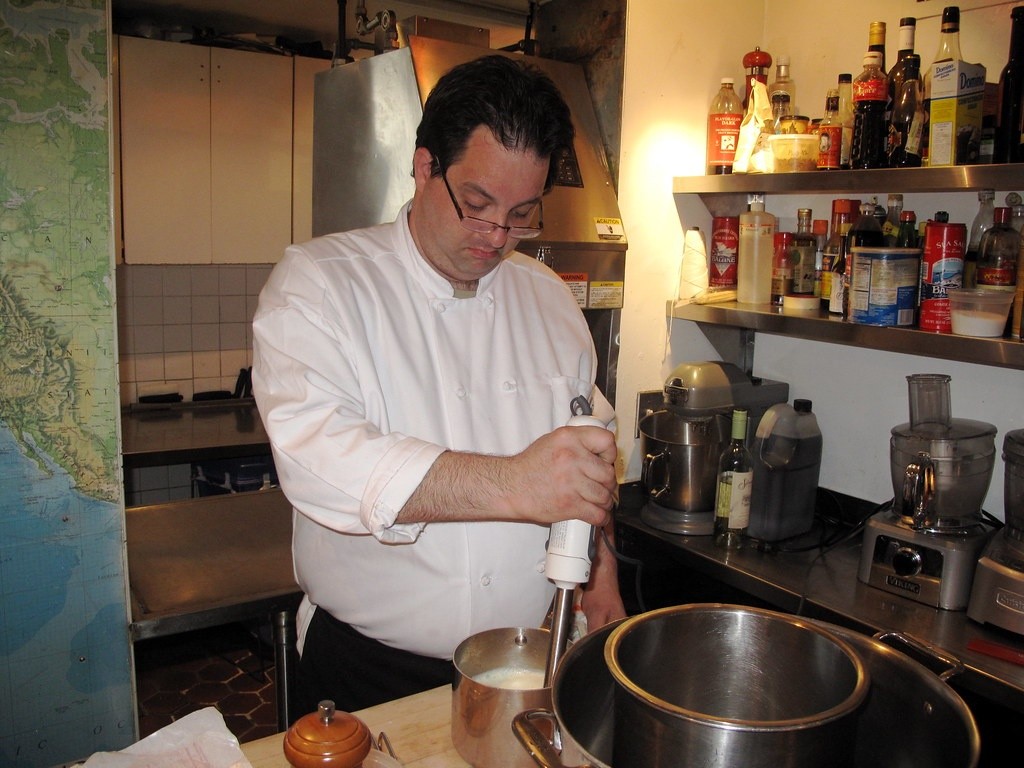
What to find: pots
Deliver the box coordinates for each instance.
[606,602,869,767]
[513,614,981,768]
[450,588,574,767]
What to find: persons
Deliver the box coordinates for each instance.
[250,53,627,727]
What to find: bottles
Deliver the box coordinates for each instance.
[747,398,823,541]
[712,408,754,550]
[283,699,372,768]
[677,192,1024,327]
[707,5,1024,175]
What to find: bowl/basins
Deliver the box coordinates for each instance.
[946,288,1016,337]
[770,134,819,171]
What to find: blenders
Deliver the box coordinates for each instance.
[634,358,790,533]
[968,428,1024,637]
[856,375,998,611]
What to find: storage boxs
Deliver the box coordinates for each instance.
[928,58,987,166]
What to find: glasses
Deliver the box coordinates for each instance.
[431,154,544,238]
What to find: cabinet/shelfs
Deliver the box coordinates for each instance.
[666,162,1024,374]
[118,33,332,265]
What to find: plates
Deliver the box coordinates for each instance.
[361,748,403,768]
